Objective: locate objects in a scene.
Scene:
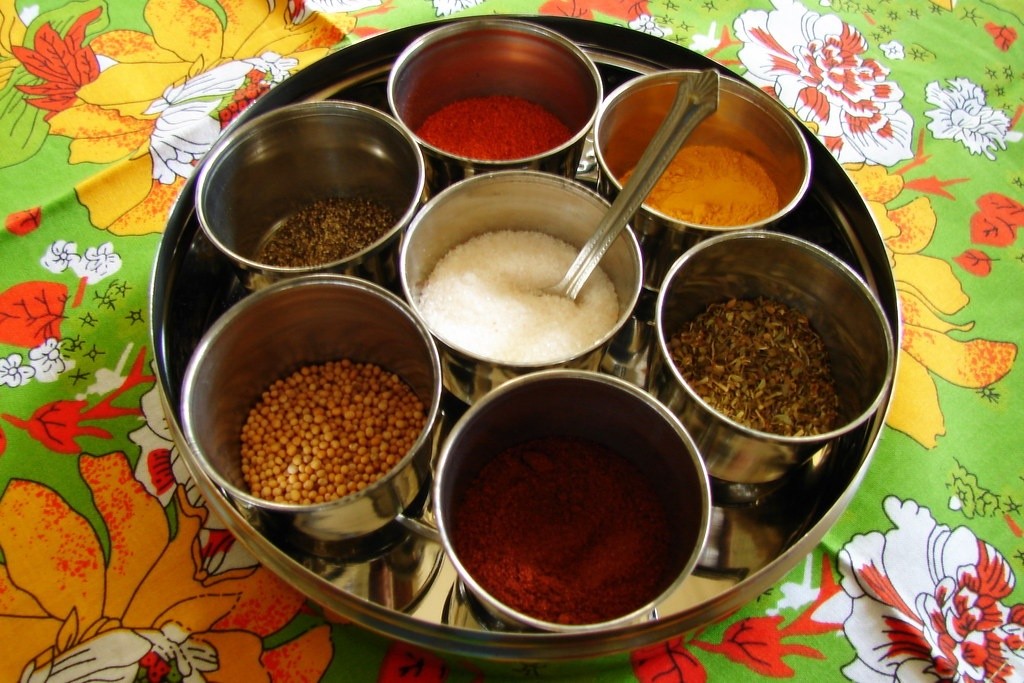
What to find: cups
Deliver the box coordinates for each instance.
[180,271,438,538]
[599,64,813,289]
[401,169,644,407]
[194,99,438,286]
[644,231,896,484]
[434,369,713,631]
[388,20,605,205]
